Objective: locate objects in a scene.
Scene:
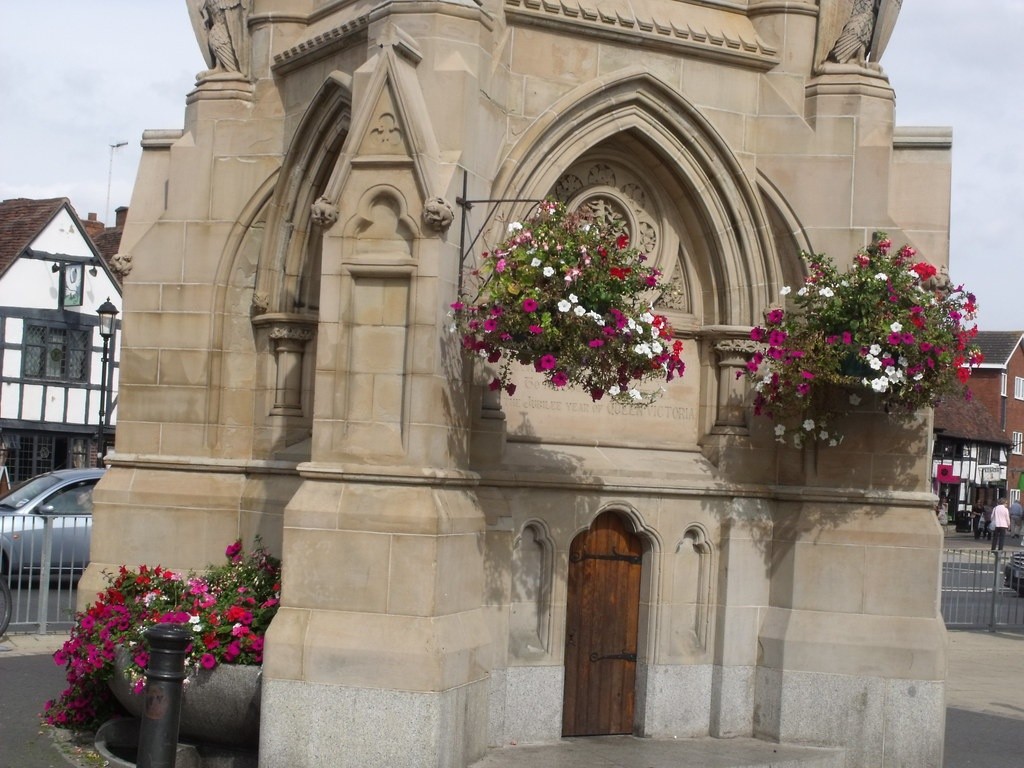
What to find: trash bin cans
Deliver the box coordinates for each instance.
[956,511,971,532]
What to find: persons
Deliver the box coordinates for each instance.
[982,499,995,542]
[989,498,1011,554]
[1009,498,1023,539]
[971,499,984,540]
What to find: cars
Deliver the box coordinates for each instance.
[0,465,112,589]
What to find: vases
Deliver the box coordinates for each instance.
[103,642,266,745]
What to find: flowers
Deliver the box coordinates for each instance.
[734,232,984,449]
[38,537,282,729]
[452,193,689,407]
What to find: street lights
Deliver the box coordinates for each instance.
[97,297,119,467]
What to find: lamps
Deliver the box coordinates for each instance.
[50,255,100,278]
